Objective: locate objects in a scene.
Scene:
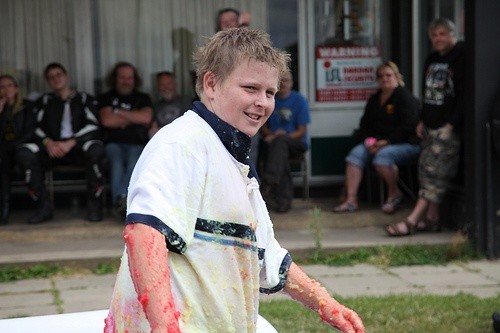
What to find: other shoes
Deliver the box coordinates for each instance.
[27,193,53,223]
[265,195,291,213]
[88,198,102,221]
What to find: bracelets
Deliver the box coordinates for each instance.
[111,106,116,113]
[239,23,249,27]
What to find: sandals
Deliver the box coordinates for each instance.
[332,202,359,212]
[381,193,406,214]
[383,217,442,236]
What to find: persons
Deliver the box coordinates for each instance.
[103,29,364,333]
[383,19,474,236]
[331,61,423,213]
[0,9,310,225]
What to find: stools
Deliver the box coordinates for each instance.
[3,164,109,217]
[366,158,418,207]
[290,149,310,200]
